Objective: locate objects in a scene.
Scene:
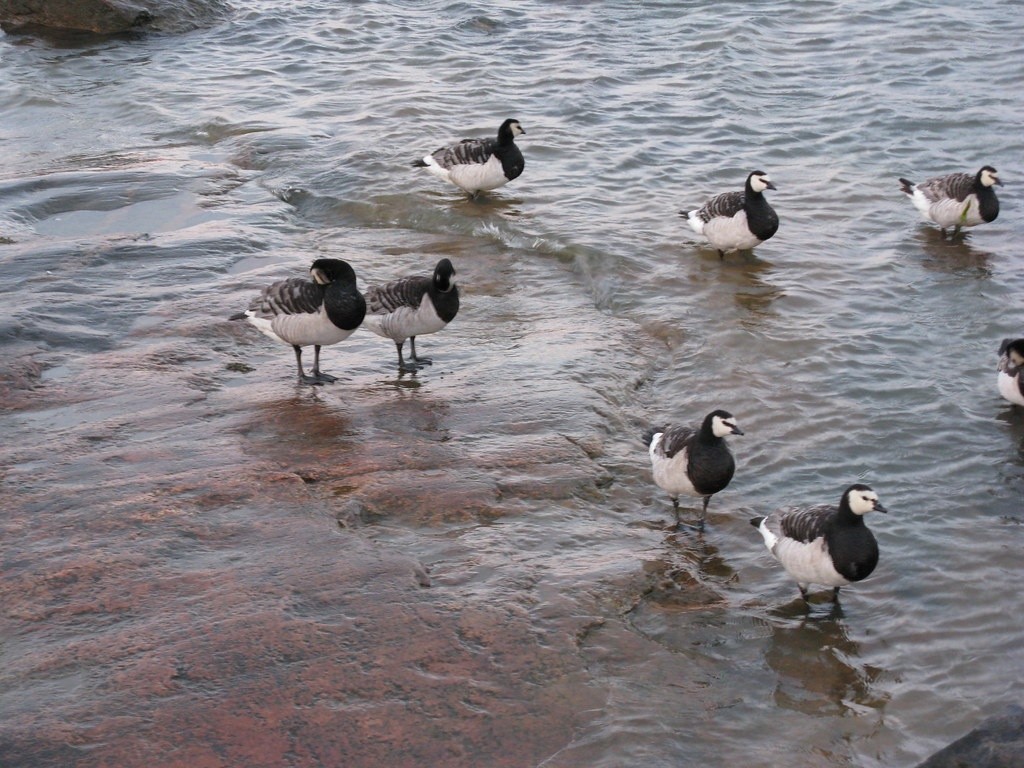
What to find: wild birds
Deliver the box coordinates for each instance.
[749,483,890,601]
[408,118,527,202]
[243,258,368,386]
[676,168,780,264]
[996,337,1024,408]
[898,165,1005,238]
[640,409,746,512]
[363,257,460,372]
